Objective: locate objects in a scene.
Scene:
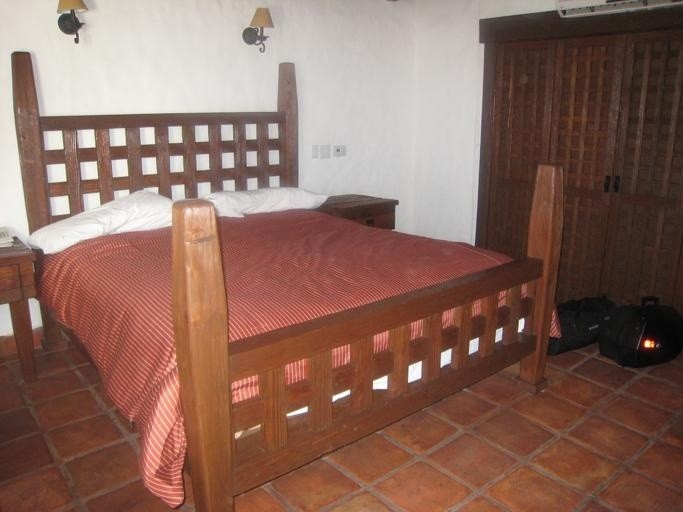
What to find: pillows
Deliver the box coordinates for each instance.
[206,187,333,218]
[26,190,174,256]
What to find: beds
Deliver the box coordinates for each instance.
[11,51,566,512]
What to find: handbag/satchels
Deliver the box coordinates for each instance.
[598,296,683,367]
[547,294,617,356]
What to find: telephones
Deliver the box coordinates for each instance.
[0,227,13,247]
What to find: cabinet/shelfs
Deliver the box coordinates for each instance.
[475,6,683,323]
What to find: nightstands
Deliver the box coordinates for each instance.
[319,194,399,229]
[0,237,37,383]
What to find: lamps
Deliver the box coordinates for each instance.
[242,8,274,53]
[57,0,88,43]
